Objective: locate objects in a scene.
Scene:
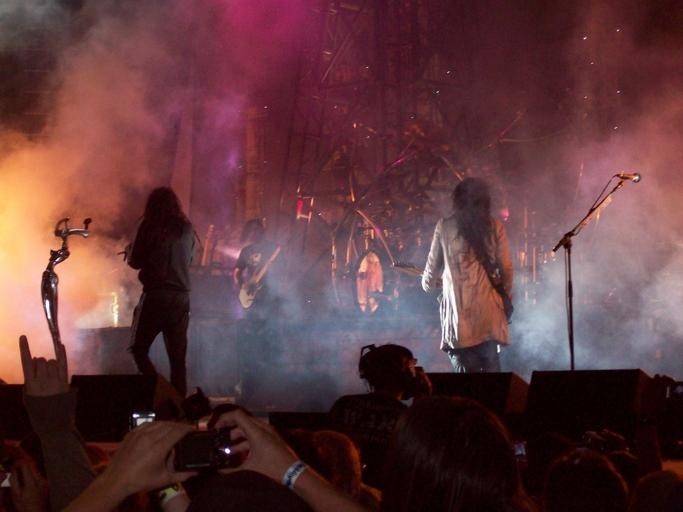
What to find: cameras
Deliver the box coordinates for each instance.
[174,430,239,471]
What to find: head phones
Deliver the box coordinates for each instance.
[358,343,417,400]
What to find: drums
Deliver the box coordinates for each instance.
[353,251,396,316]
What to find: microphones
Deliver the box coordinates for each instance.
[617,173,641,183]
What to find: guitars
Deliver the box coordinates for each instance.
[239,245,281,308]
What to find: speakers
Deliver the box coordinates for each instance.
[69,373,186,444]
[0,384,32,440]
[527,368,662,467]
[425,372,529,442]
[76,264,236,395]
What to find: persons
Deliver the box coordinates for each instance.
[234,217,283,328]
[124,182,204,397]
[419,175,514,375]
[0,332,681,509]
